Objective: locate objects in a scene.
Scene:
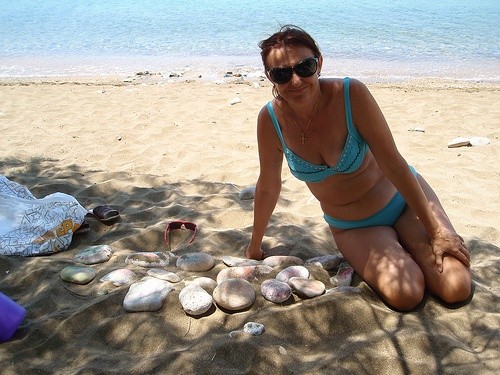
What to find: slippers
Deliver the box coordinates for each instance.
[86,206,120,223]
[73,221,91,234]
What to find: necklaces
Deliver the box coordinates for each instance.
[290,90,320,145]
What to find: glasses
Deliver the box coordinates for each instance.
[267,57,319,85]
[164,221,197,244]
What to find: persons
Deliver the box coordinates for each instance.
[245,27,473,312]
